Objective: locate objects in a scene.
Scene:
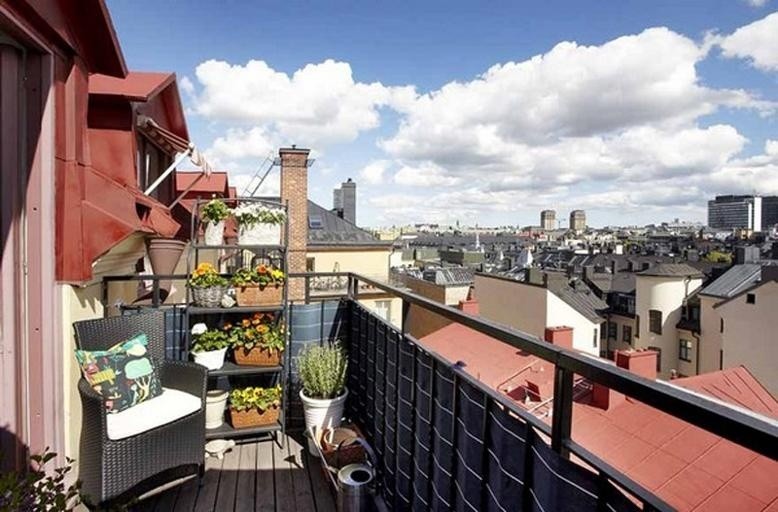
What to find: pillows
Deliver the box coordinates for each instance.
[74,329,164,413]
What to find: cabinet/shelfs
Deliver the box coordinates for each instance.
[185,200,290,451]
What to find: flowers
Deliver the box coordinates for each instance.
[229,383,283,412]
[230,200,287,230]
[190,321,227,351]
[185,262,229,289]
[223,313,285,355]
[199,199,230,224]
[228,265,285,287]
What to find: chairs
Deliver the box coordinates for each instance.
[72,311,208,511]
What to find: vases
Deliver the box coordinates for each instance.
[205,389,229,430]
[324,428,358,450]
[203,219,224,245]
[191,286,222,308]
[190,346,227,370]
[235,286,282,306]
[235,219,281,245]
[234,345,280,366]
[228,404,280,428]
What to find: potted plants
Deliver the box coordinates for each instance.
[296,340,349,458]
